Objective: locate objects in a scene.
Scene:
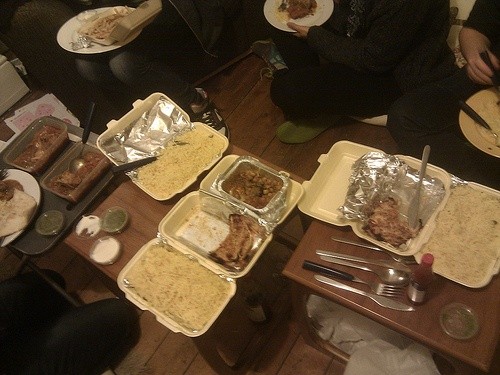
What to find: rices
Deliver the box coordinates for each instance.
[135,129,225,199]
[419,185,500,285]
[126,245,233,332]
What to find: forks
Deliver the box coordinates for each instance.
[301,260,406,297]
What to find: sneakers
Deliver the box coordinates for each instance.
[277,112,341,143]
[189,88,230,142]
[252,40,288,71]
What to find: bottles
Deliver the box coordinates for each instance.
[406,253,434,307]
[241,274,272,325]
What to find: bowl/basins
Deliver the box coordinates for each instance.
[39,139,113,204]
[3,115,69,172]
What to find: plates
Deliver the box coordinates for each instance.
[101,206,129,233]
[36,209,65,236]
[460,89,500,159]
[0,169,41,249]
[264,0,334,32]
[56,6,143,54]
[89,236,122,264]
[439,302,480,340]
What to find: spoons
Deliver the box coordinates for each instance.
[318,256,411,285]
[69,102,98,174]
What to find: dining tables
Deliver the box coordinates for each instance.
[0,89,498,375]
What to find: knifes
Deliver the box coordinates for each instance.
[313,273,415,312]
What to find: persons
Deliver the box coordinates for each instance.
[386,0,500,186]
[0,269,140,375]
[0,0,269,144]
[266,0,449,145]
